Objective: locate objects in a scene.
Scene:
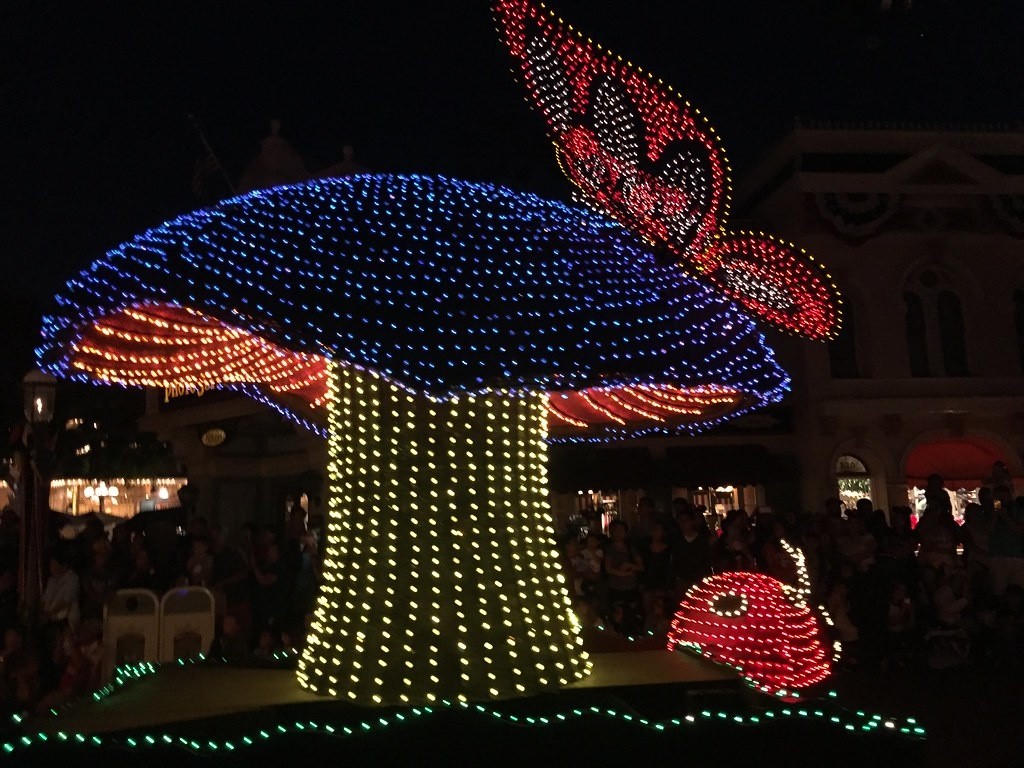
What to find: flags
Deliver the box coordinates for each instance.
[189,128,221,194]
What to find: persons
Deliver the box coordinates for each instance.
[558,461,1024,670]
[0,485,325,719]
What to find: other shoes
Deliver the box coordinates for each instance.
[879,653,889,664]
[896,656,907,667]
